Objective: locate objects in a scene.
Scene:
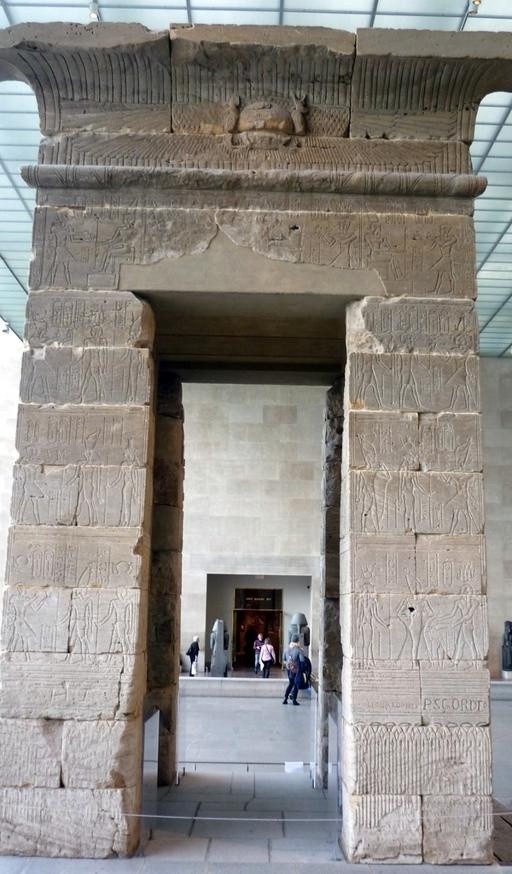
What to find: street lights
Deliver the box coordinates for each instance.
[269,653,274,665]
[191,659,198,675]
[186,648,192,655]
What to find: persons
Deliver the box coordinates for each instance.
[223,624,277,677]
[189,635,199,676]
[281,633,305,705]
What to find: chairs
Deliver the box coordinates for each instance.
[281,694,301,705]
